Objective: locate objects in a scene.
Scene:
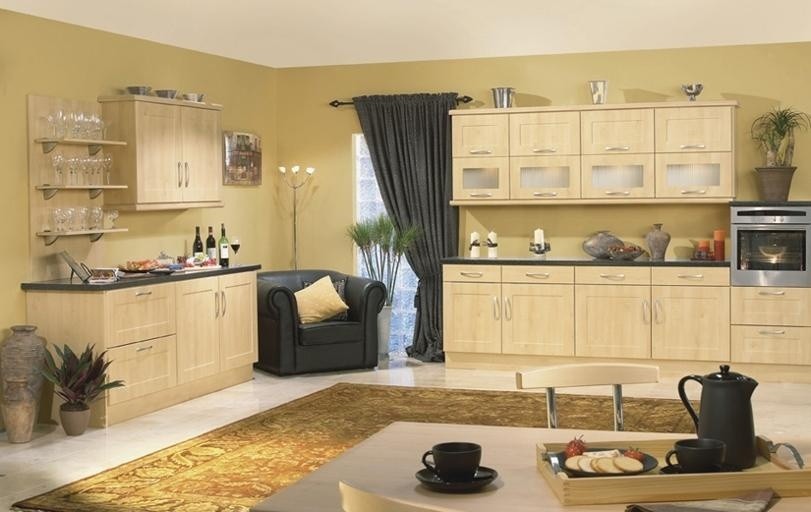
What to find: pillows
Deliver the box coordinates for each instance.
[293,273,350,325]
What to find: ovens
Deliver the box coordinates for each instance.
[729,206,811,286]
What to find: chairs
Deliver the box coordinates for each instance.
[514,362,661,431]
[336,478,452,512]
[256,269,388,377]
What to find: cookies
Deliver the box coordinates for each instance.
[566,455,643,474]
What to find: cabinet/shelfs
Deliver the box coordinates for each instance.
[21,281,178,408]
[34,136,129,245]
[582,101,740,205]
[575,266,732,363]
[449,105,582,205]
[178,268,258,386]
[98,94,224,211]
[730,286,811,367]
[443,264,576,357]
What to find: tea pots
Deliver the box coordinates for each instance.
[678,364,759,469]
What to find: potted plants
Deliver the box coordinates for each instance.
[751,103,811,202]
[350,216,427,360]
[37,342,126,434]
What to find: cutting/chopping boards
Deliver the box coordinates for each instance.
[176,265,223,272]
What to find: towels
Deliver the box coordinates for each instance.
[302,276,348,322]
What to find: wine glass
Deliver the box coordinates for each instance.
[682,84,703,101]
[47,155,113,186]
[230,240,241,265]
[48,205,119,230]
[44,109,105,140]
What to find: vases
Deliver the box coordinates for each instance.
[0,323,47,413]
[648,222,672,262]
[1,375,38,443]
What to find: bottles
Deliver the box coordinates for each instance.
[713,228,726,261]
[646,223,671,263]
[204,227,216,262]
[193,225,204,257]
[218,223,228,267]
[697,239,709,253]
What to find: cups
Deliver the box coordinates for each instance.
[666,439,727,470]
[492,87,514,110]
[421,442,481,481]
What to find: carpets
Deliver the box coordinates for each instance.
[10,381,704,512]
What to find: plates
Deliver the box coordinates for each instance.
[416,465,499,492]
[660,462,744,474]
[546,448,657,476]
[115,268,160,273]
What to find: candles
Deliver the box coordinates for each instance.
[469,232,481,256]
[535,226,546,252]
[487,228,499,257]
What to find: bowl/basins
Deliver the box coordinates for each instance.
[154,89,176,99]
[601,247,645,260]
[182,92,203,102]
[126,85,152,94]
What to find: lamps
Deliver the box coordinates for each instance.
[279,164,317,270]
